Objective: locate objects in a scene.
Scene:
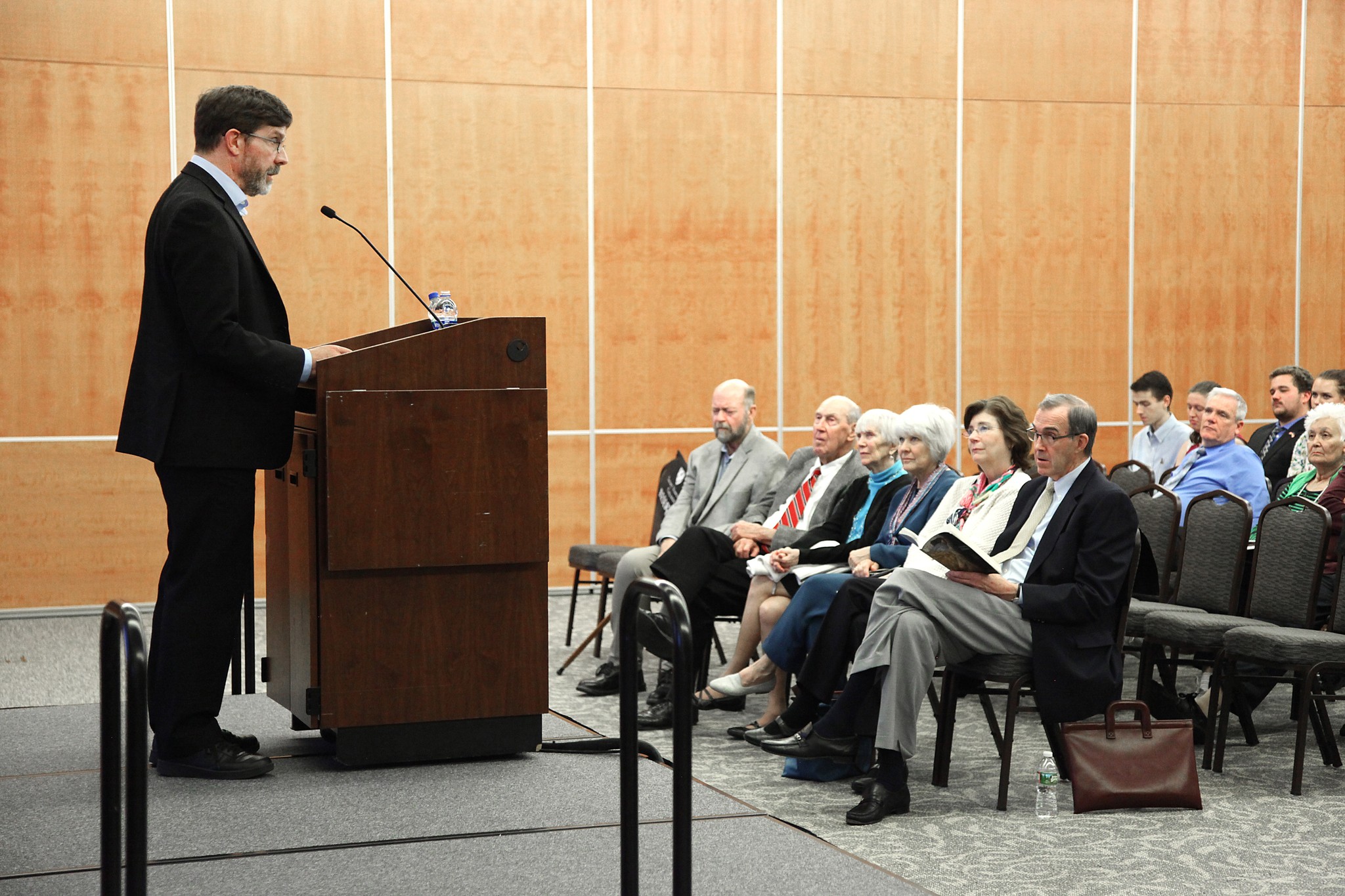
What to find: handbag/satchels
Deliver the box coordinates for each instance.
[1056,696,1203,814]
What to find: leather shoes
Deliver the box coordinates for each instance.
[576,661,647,696]
[647,668,673,703]
[852,763,883,791]
[158,739,273,778]
[759,721,859,763]
[744,715,796,746]
[846,780,910,826]
[150,728,260,766]
[636,699,700,731]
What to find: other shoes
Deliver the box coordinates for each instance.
[1143,677,1208,744]
[709,667,778,696]
[727,720,759,740]
[693,687,745,712]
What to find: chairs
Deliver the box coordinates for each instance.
[565,451,1345,811]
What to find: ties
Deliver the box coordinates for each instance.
[991,481,1055,562]
[719,454,731,480]
[762,468,821,553]
[1259,426,1284,461]
[1153,448,1206,499]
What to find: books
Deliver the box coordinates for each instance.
[898,522,1001,575]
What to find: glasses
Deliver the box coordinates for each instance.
[1025,426,1084,444]
[223,128,286,153]
[962,426,1001,438]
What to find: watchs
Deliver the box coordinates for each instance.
[1010,584,1021,603]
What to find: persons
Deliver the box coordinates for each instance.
[636,395,870,732]
[1146,396,1345,747]
[1286,369,1345,478]
[1248,365,1314,491]
[845,392,1139,826]
[1135,387,1271,602]
[744,404,963,747]
[575,379,789,703]
[745,397,1032,744]
[1175,381,1245,467]
[1128,370,1195,495]
[689,407,906,737]
[115,86,355,781]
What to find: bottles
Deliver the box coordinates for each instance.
[1035,751,1058,818]
[434,291,458,330]
[428,292,441,330]
